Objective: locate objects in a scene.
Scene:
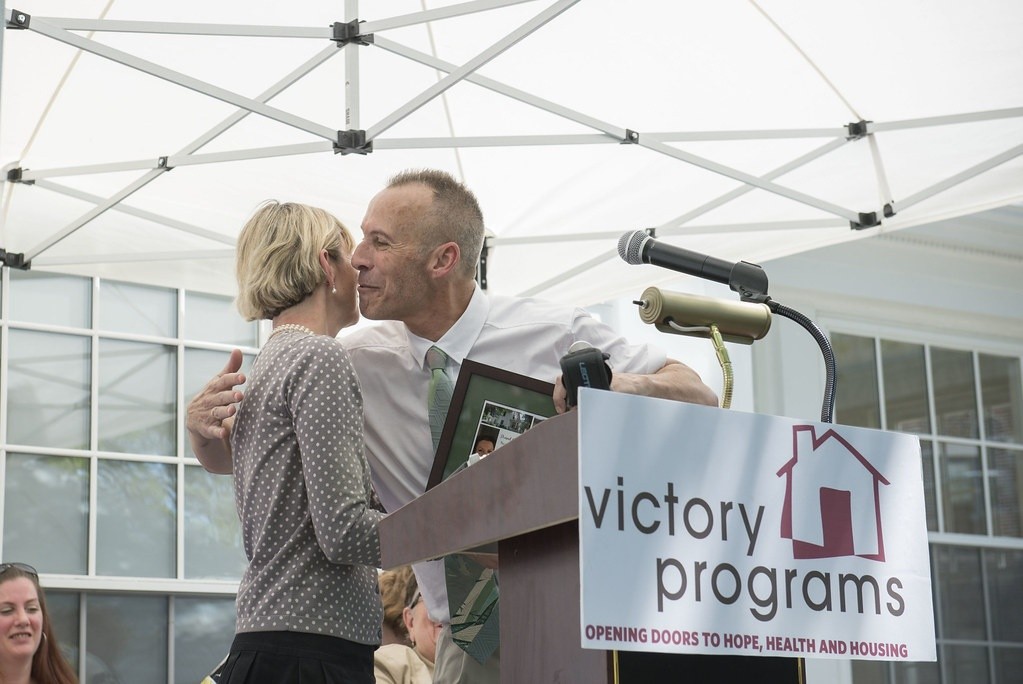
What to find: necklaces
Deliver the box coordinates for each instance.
[266,324,316,341]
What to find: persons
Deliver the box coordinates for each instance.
[374,563,443,684]
[473,435,496,456]
[0,562,80,684]
[184,169,718,684]
[220,199,498,684]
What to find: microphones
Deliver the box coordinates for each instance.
[617,230,768,295]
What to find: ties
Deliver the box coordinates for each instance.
[426,346,500,667]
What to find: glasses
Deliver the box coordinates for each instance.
[0,563,39,583]
